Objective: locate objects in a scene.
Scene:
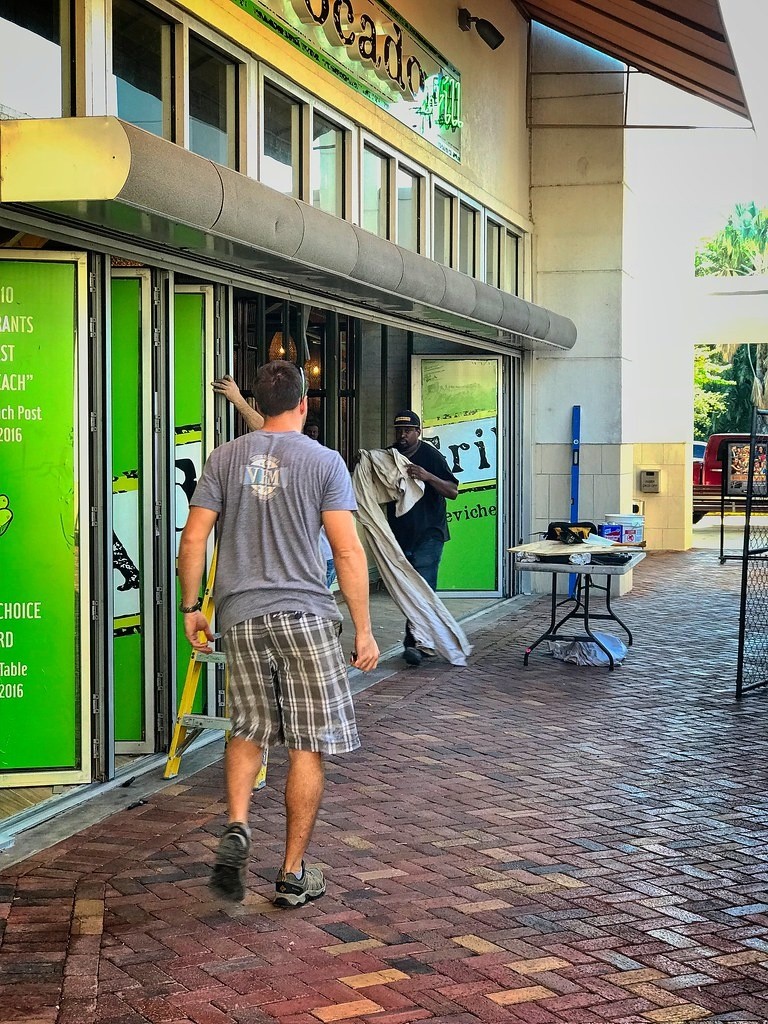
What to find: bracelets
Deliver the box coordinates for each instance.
[179,597,203,614]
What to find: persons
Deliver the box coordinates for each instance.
[208,374,337,589]
[177,360,382,911]
[304,420,321,442]
[354,410,459,667]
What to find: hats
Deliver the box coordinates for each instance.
[393,410,421,430]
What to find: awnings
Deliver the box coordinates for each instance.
[511,0,758,134]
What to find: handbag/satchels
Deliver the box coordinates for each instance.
[543,521,598,545]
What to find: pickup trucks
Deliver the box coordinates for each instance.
[693,433,768,526]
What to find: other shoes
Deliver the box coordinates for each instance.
[404,649,422,666]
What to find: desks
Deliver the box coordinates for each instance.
[515,552,647,667]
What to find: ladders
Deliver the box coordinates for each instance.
[160,531,269,792]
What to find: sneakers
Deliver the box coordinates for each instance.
[208,822,253,904]
[272,859,326,908]
